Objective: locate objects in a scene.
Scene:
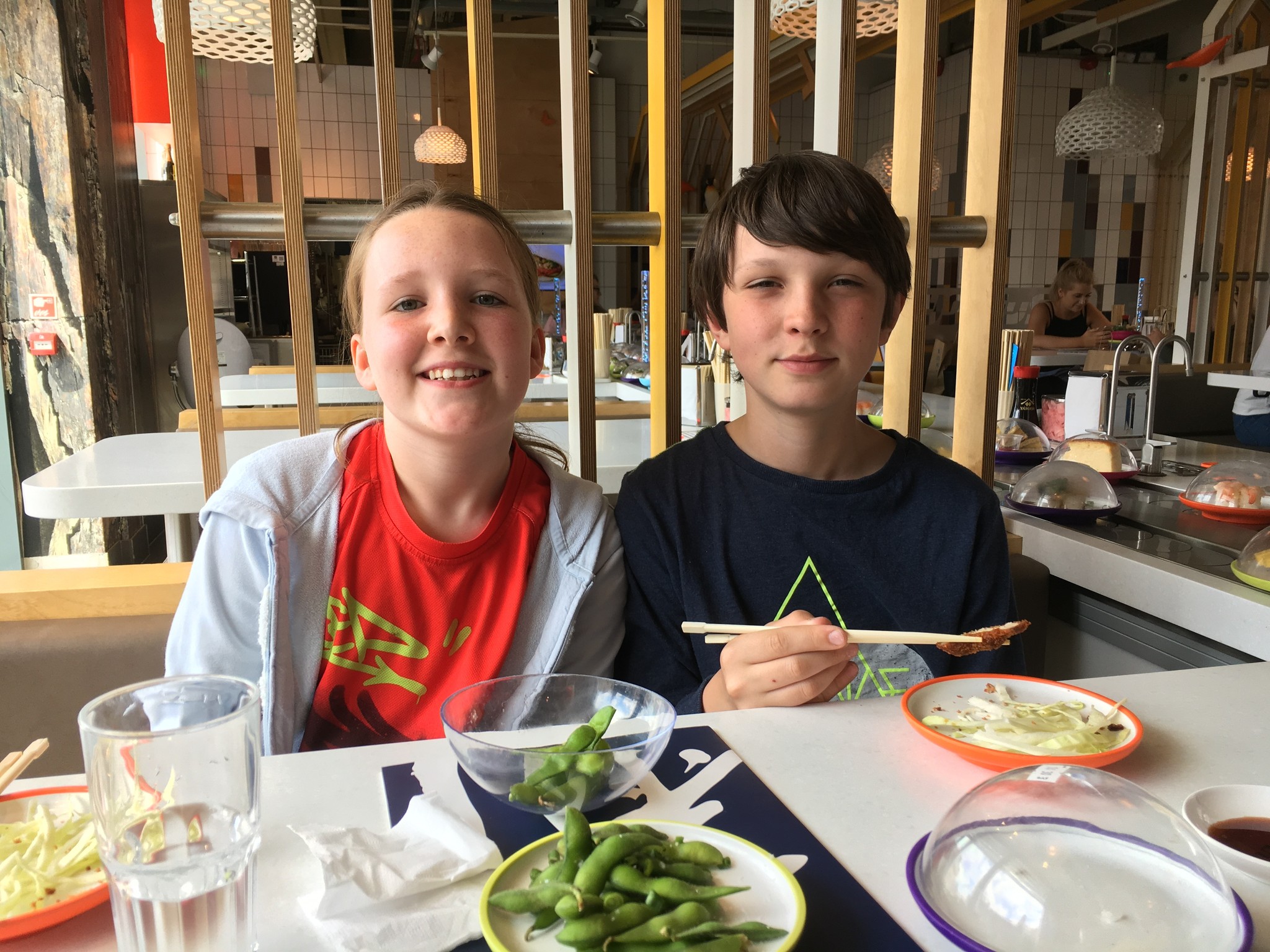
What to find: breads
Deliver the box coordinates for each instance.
[1063,437,1122,472]
[999,422,1044,452]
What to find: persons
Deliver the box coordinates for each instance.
[1027,257,1110,406]
[542,273,605,338]
[1144,280,1245,365]
[1231,323,1270,452]
[614,150,1018,717]
[137,182,623,757]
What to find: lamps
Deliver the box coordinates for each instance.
[588,39,603,75]
[413,0,467,165]
[1092,27,1115,54]
[421,36,443,71]
[1225,147,1270,182]
[771,1,899,40]
[1055,55,1163,159]
[863,140,941,195]
[626,0,648,27]
[152,0,316,65]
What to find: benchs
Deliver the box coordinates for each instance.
[1104,363,1270,453]
[0,560,195,778]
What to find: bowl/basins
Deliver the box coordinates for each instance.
[439,673,676,813]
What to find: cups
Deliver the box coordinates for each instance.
[77,674,266,952]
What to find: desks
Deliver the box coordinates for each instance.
[1031,348,1086,366]
[0,660,1267,952]
[1207,369,1270,392]
[218,376,618,409]
[21,418,696,562]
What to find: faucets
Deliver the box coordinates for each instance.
[1136,333,1194,478]
[1104,334,1156,437]
[623,310,642,345]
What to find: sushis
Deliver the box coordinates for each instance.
[1213,479,1266,509]
[1253,549,1270,582]
[1018,477,1084,510]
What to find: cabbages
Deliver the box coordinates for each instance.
[0,768,178,922]
[921,685,1130,755]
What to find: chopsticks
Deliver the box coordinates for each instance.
[592,306,636,380]
[997,328,1035,420]
[682,618,1014,652]
[696,326,731,423]
[0,736,49,798]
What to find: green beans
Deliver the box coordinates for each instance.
[487,805,790,952]
[509,706,615,808]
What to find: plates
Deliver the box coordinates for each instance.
[993,448,1055,465]
[856,414,874,426]
[1178,493,1270,524]
[868,413,936,431]
[1003,493,1124,526]
[1042,458,1140,480]
[902,673,1144,774]
[1180,783,1269,885]
[605,345,652,391]
[0,787,114,942]
[483,817,806,952]
[907,763,1239,952]
[1108,331,1142,350]
[1230,559,1270,594]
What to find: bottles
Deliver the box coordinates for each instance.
[1003,364,1041,440]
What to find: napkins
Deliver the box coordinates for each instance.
[286,795,503,952]
